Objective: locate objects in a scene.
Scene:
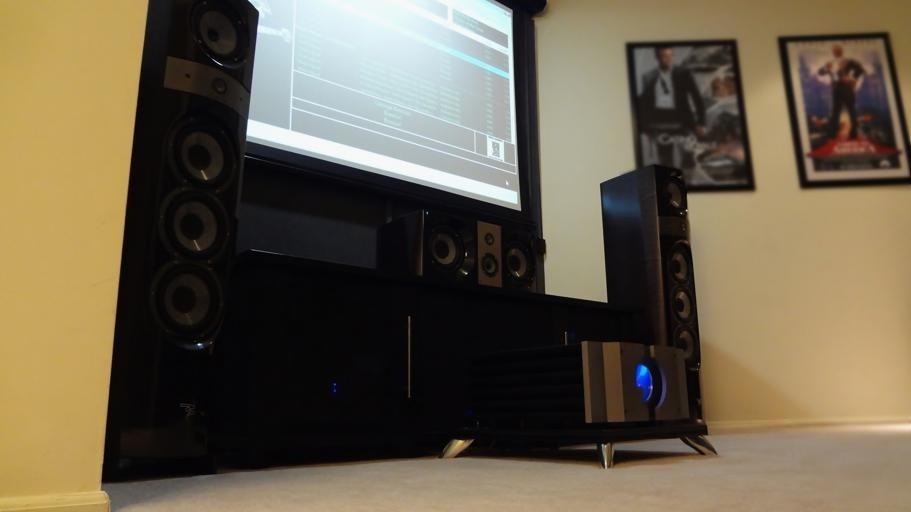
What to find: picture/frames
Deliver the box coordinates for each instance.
[625,39,755,192]
[776,31,911,189]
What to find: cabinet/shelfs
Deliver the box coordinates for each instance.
[203,246,643,475]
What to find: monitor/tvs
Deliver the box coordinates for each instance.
[243,0,537,231]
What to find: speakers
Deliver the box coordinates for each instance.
[104,0,259,484]
[378,208,533,291]
[600,165,703,418]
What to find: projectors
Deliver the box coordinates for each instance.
[438,338,718,469]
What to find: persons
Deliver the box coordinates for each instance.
[643,46,706,167]
[818,44,866,142]
